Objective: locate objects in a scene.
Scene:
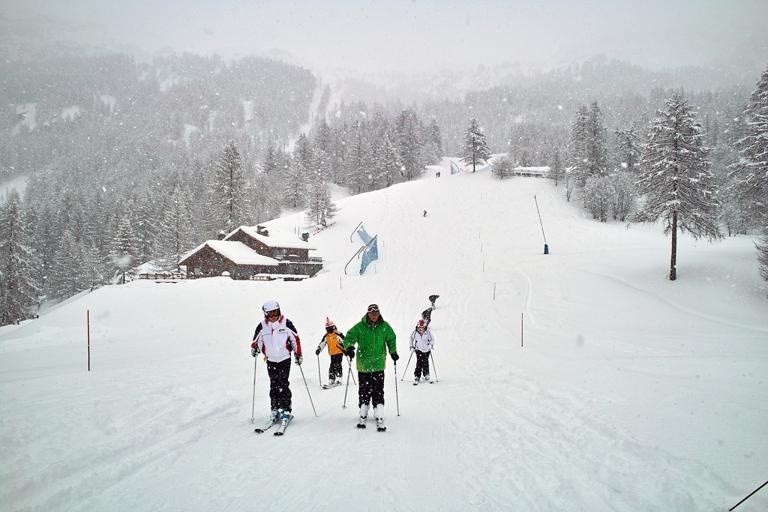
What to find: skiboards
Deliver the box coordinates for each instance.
[357,408,387,431]
[412,377,436,386]
[324,380,343,389]
[254,414,294,436]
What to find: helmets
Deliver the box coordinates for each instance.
[417,319,427,328]
[325,321,336,330]
[263,299,280,312]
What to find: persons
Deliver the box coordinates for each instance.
[342,303,399,423]
[250,300,304,423]
[410,320,434,386]
[315,316,353,386]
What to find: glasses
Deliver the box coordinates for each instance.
[268,310,280,318]
[419,326,425,329]
[368,306,379,312]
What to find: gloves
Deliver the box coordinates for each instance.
[390,352,399,361]
[250,346,261,357]
[316,349,320,355]
[294,352,304,365]
[346,346,356,358]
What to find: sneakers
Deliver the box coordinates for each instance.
[360,403,370,419]
[270,410,280,421]
[425,374,430,380]
[336,377,343,384]
[280,411,290,421]
[414,376,420,382]
[373,403,386,422]
[328,378,336,385]
